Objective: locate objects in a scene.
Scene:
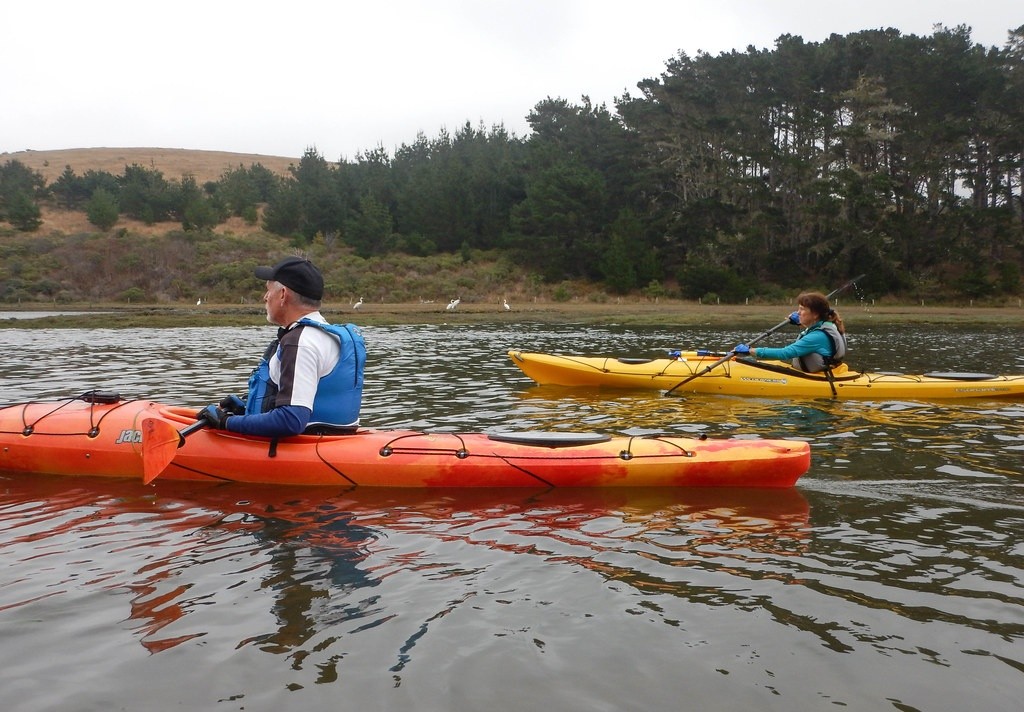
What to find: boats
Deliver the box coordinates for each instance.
[1,388,808,490]
[508,347,1024,398]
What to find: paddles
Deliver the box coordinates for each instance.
[140,407,232,487]
[664,272,866,396]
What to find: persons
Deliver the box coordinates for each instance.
[196,255,366,437]
[731,292,847,373]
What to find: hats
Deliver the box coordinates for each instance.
[254,256,325,300]
